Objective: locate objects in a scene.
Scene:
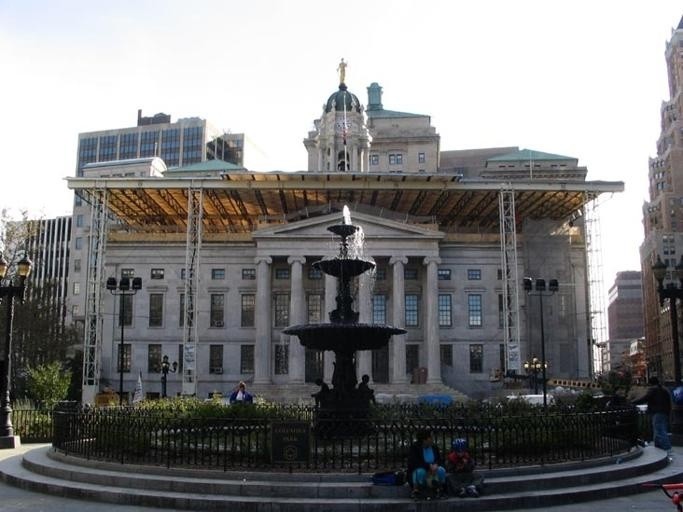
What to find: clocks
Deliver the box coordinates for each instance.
[334,116,352,132]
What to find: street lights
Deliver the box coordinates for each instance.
[103,273,143,407]
[0,248,34,451]
[595,342,607,373]
[523,353,548,395]
[153,355,178,400]
[521,275,559,407]
[650,251,683,448]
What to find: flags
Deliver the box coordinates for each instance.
[132,371,144,404]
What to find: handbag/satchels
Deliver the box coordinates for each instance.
[371,471,407,486]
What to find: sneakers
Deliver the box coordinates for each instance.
[409,484,479,500]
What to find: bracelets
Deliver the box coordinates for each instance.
[429,463,432,469]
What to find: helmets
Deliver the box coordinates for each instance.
[450,437,469,451]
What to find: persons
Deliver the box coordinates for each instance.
[336,58,348,83]
[311,376,329,407]
[229,381,254,405]
[407,429,447,502]
[444,438,484,497]
[359,374,376,405]
[632,375,672,450]
